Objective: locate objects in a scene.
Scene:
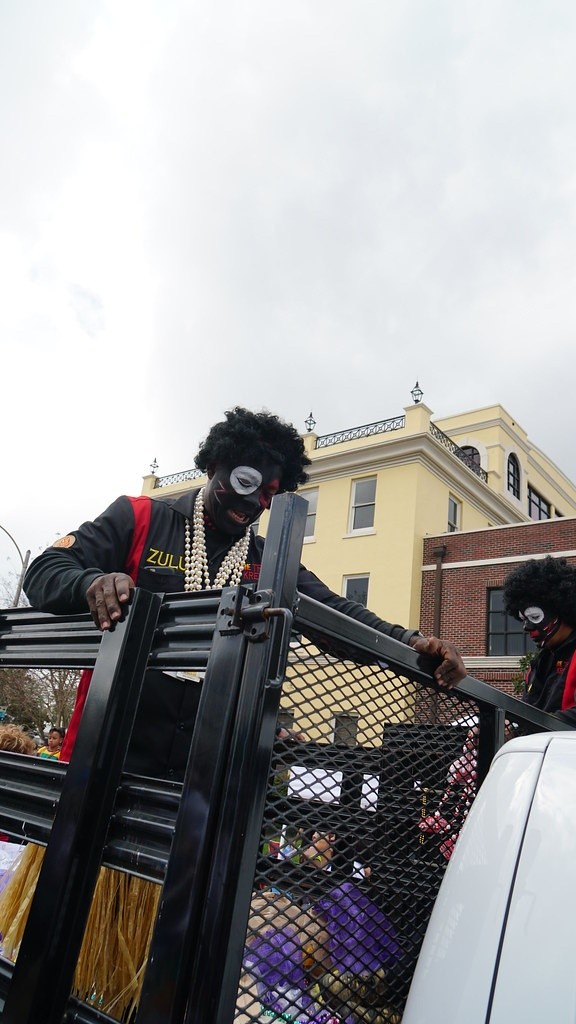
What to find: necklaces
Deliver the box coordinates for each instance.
[183,489,250,590]
[418,788,428,844]
[37,745,60,757]
[436,778,470,834]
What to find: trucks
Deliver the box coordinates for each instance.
[1,493,576,1020]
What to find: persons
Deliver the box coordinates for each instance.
[281,823,370,880]
[500,555,576,737]
[0,721,65,892]
[0,410,469,1024]
